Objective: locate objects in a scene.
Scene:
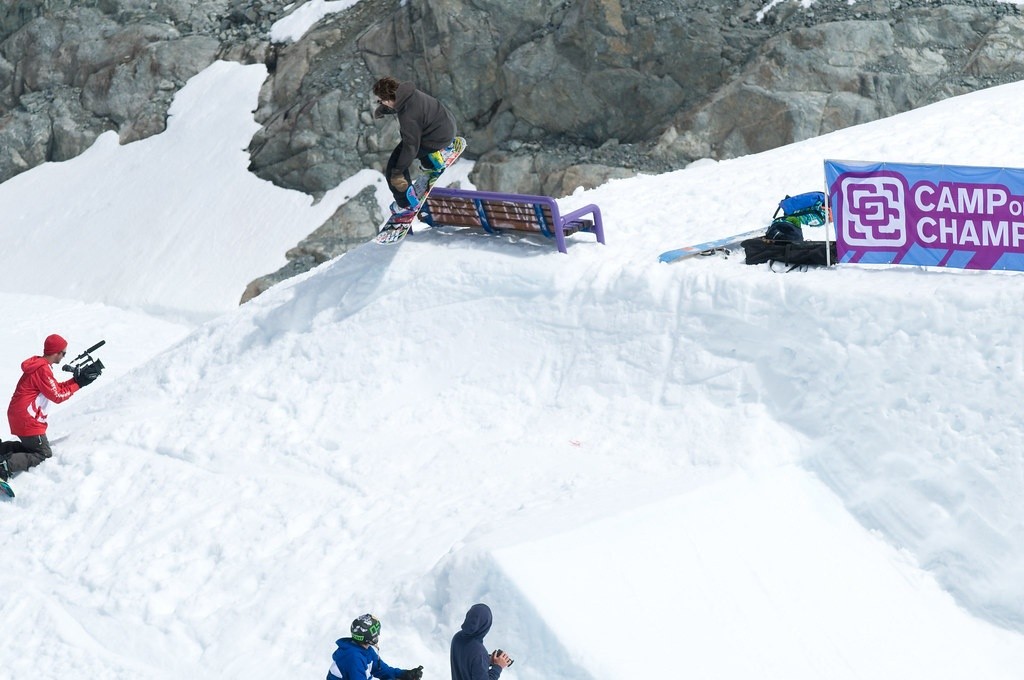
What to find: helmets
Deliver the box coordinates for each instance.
[350,614,381,644]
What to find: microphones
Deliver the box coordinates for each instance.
[79,341,105,359]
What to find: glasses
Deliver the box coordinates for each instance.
[61,351,66,357]
[377,99,384,104]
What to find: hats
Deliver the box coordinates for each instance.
[43,334,68,356]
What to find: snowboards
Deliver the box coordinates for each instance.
[656,226,772,265]
[375,136,466,247]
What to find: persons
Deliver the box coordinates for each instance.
[372,78,457,215]
[451,604,511,680]
[327,614,423,680]
[0,334,98,483]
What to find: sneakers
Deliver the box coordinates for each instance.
[418,164,444,173]
[0,462,11,482]
[389,201,420,214]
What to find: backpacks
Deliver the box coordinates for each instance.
[740,238,838,274]
[764,221,803,242]
[771,191,835,227]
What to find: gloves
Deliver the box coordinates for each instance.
[78,374,97,388]
[390,174,409,193]
[404,665,423,680]
[374,105,396,119]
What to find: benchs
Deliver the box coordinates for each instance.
[416,187,605,253]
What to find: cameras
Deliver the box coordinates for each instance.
[493,649,514,667]
